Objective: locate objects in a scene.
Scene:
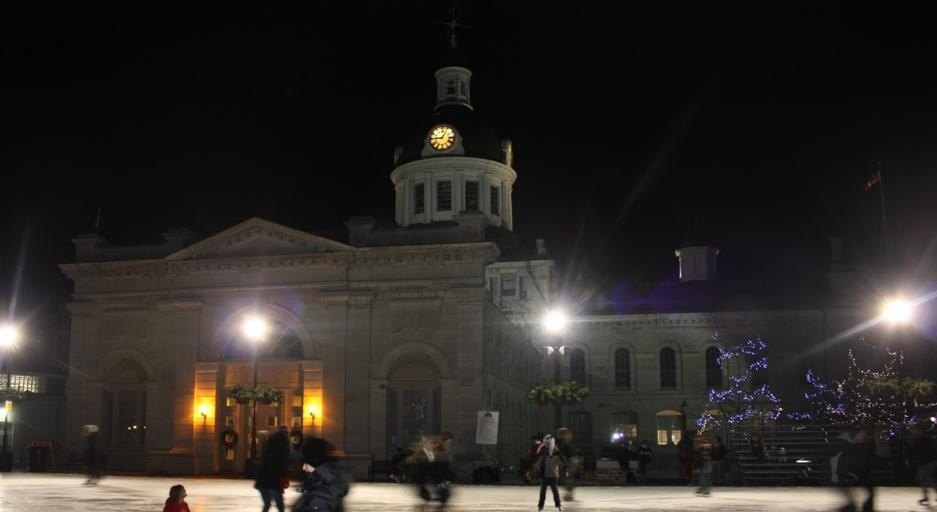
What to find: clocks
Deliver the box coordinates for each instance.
[429,126,455,151]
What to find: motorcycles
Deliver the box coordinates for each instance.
[297,463,336,512]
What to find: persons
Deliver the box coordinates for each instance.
[526,433,568,512]
[710,438,726,485]
[163,484,191,512]
[556,427,578,501]
[406,431,456,512]
[252,423,348,512]
[677,429,697,486]
[81,423,102,486]
[905,421,937,503]
[696,444,714,495]
[633,440,654,474]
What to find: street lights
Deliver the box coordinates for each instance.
[880,296,915,484]
[240,313,274,477]
[1,324,21,472]
[539,308,572,449]
